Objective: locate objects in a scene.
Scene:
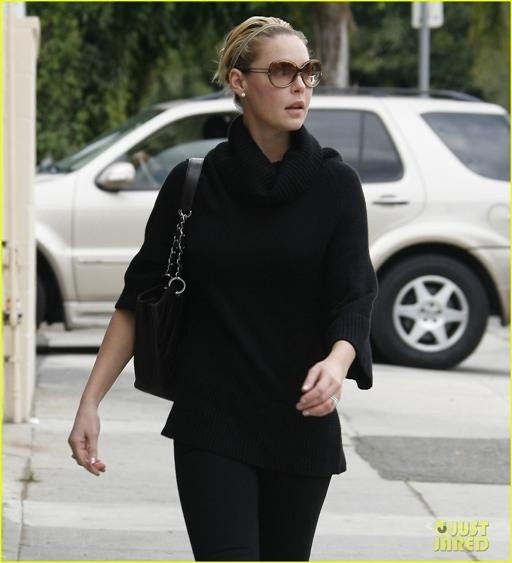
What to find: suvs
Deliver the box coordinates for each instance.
[32,88,511,367]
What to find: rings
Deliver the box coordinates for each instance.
[329,395,338,404]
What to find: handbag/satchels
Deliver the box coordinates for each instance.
[134,271,186,400]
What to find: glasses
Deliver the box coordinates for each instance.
[238,57,324,91]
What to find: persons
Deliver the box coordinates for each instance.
[67,14,383,560]
